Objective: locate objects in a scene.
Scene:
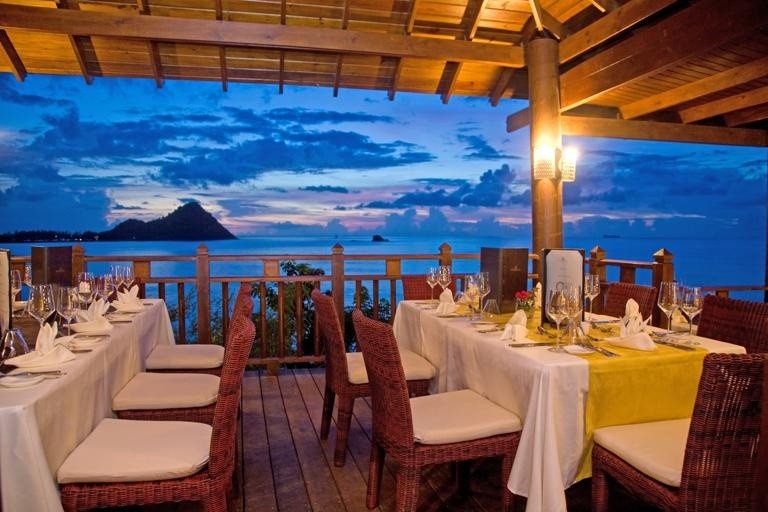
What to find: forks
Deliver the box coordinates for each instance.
[580,336,616,357]
[651,328,685,336]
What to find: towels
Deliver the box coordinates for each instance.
[64,298,116,335]
[435,288,457,316]
[619,296,645,326]
[502,305,530,340]
[3,319,77,370]
[108,282,144,308]
[606,329,659,352]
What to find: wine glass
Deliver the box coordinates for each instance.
[545,285,582,352]
[438,265,452,291]
[680,286,704,344]
[426,266,439,303]
[25,264,135,344]
[10,269,22,317]
[584,274,601,322]
[658,281,681,337]
[464,272,492,322]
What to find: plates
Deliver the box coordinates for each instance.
[1,371,45,389]
[563,345,595,355]
[69,346,93,352]
[593,314,619,322]
[438,312,459,317]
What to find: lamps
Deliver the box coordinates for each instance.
[533,133,579,184]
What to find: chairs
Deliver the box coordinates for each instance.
[144,281,253,421]
[696,292,767,352]
[398,272,460,305]
[309,284,436,467]
[53,314,258,512]
[109,291,254,501]
[590,351,767,512]
[345,304,523,511]
[600,280,657,327]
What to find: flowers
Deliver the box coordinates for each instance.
[515,287,535,307]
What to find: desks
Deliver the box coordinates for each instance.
[0,296,176,512]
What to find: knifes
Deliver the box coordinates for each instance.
[1,369,61,378]
[509,341,561,348]
[653,339,695,351]
[479,327,504,333]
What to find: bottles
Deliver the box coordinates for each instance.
[453,292,464,305]
[0,326,30,375]
[480,298,500,322]
